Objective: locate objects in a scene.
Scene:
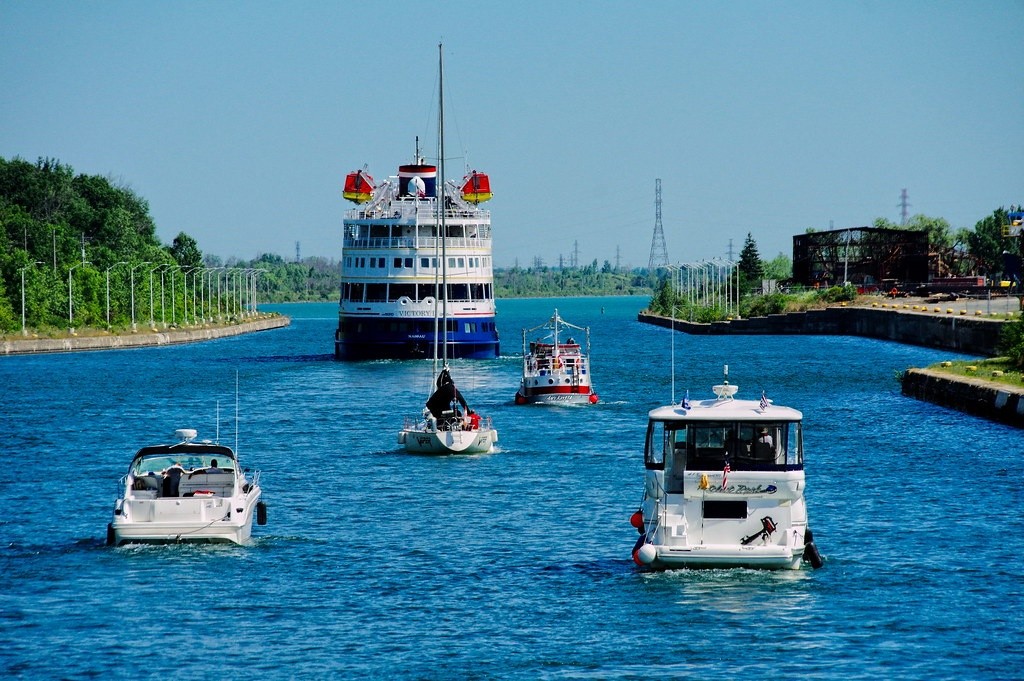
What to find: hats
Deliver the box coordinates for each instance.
[761,428,768,433]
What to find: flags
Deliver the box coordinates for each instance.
[759,394,770,411]
[680,395,691,410]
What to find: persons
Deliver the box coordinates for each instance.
[756,427,773,448]
[206,458,226,474]
[467,409,483,429]
[167,461,186,497]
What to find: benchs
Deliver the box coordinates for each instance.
[179,473,235,497]
[696,447,727,470]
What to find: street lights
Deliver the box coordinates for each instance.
[666,259,740,320]
[106,262,129,331]
[69,262,90,335]
[172,265,191,326]
[150,264,168,328]
[162,266,181,328]
[226,268,240,321]
[20,262,43,336]
[217,267,232,320]
[209,267,225,323]
[130,262,152,329]
[193,267,209,324]
[202,268,219,324]
[233,268,267,319]
[184,267,200,325]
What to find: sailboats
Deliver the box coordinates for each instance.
[398,44,498,455]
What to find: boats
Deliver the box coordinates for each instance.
[631,302,823,571]
[334,136,501,362]
[106,370,267,546]
[514,308,598,406]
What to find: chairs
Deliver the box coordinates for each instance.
[131,472,158,500]
[754,443,775,465]
[675,441,694,461]
